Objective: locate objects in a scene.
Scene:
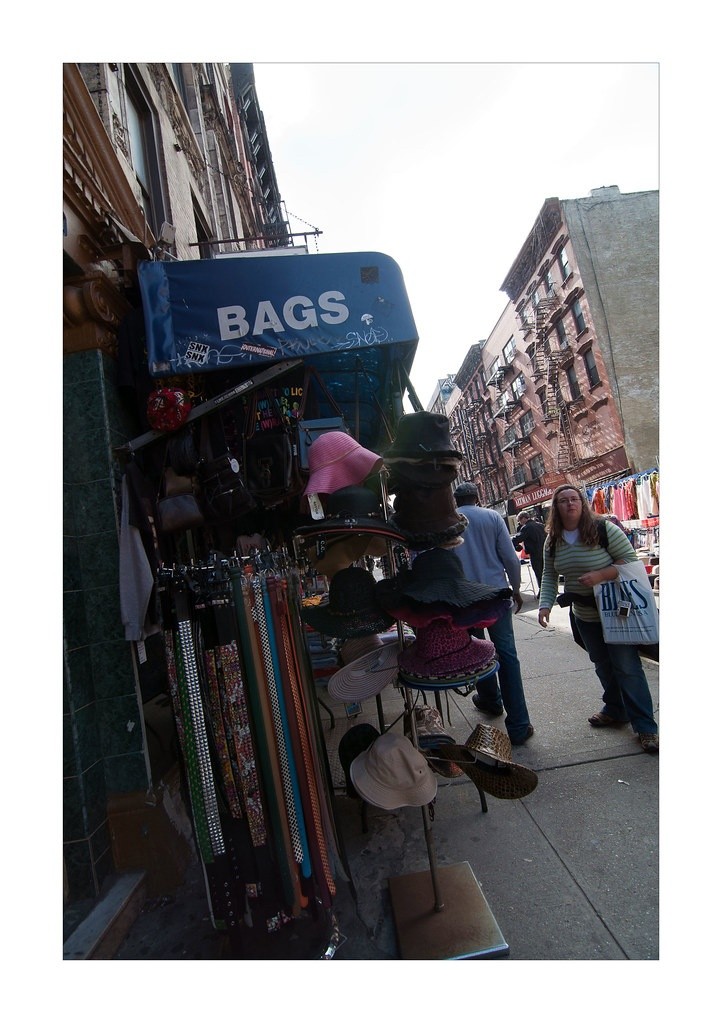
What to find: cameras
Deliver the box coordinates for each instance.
[616,600,631,619]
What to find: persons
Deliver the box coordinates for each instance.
[510,511,548,599]
[449,482,533,747]
[536,485,660,755]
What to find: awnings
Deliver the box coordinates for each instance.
[137,252,420,451]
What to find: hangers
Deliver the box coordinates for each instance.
[585,466,659,494]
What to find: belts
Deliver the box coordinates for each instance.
[147,546,351,955]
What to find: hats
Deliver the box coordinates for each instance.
[289,412,538,811]
[145,387,191,432]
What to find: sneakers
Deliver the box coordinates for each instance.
[589,712,615,728]
[639,731,659,752]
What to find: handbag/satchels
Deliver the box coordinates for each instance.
[150,349,424,534]
[592,561,658,646]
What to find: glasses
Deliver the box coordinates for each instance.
[558,497,582,505]
